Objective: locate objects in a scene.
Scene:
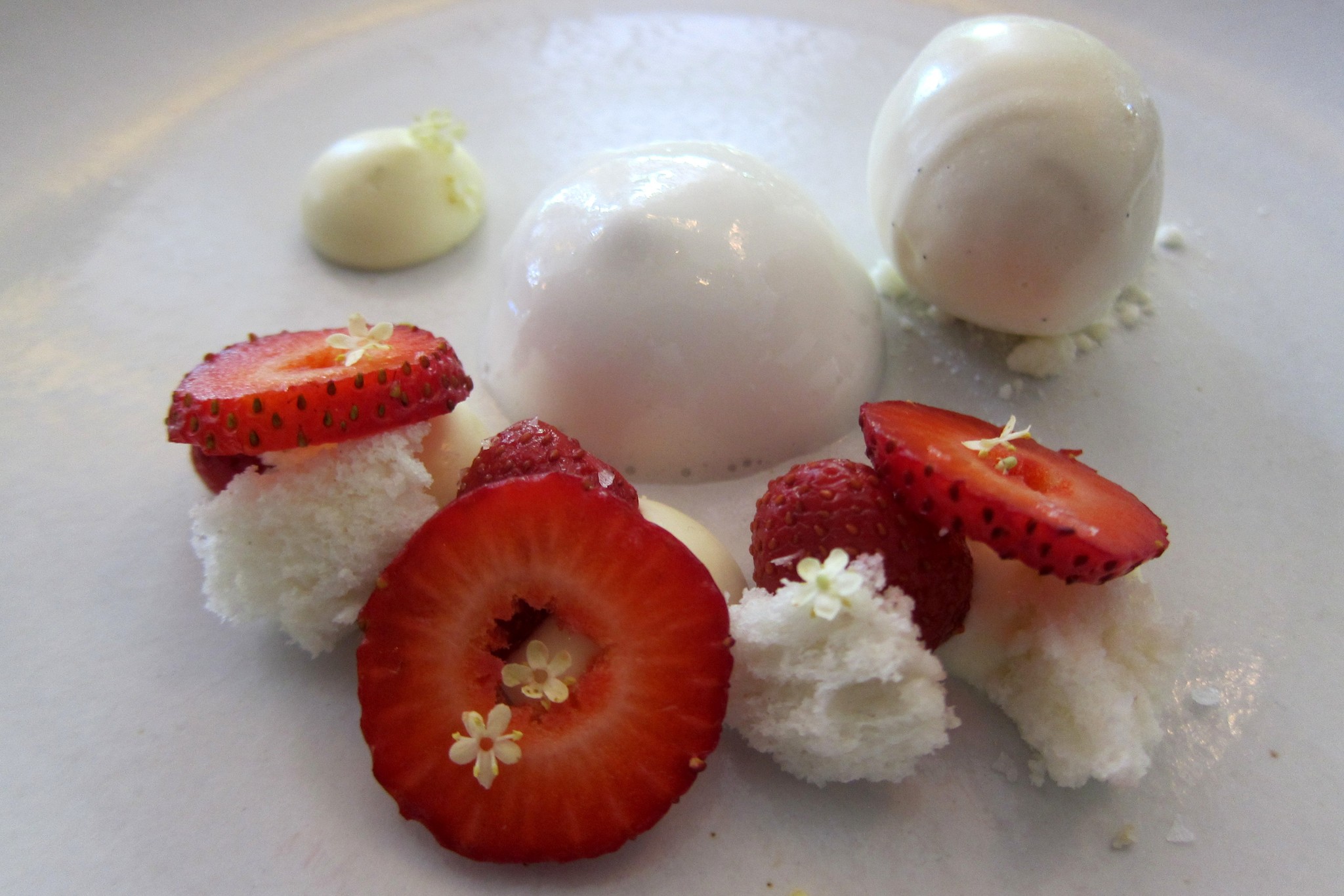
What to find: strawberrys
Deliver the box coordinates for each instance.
[164,320,474,497]
[356,414,734,865]
[749,399,1170,652]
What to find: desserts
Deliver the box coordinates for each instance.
[303,125,484,271]
[478,142,884,484]
[872,12,1182,377]
